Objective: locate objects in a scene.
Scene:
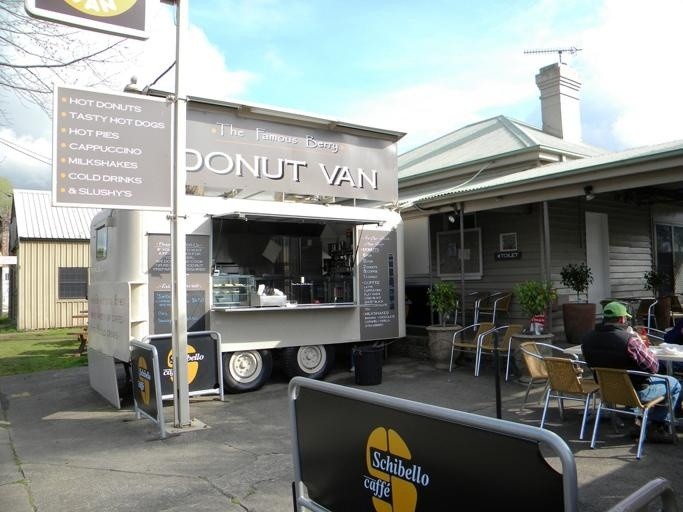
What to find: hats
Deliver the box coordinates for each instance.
[603,302,632,319]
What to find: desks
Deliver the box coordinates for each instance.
[72,310,88,331]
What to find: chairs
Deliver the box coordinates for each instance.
[448,291,683,460]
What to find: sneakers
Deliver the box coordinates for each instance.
[631,417,680,444]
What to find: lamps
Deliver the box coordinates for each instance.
[105,209,114,227]
[584,186,594,201]
[448,205,458,223]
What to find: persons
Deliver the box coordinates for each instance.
[580,300,683,445]
[659,317,683,430]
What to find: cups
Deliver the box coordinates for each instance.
[300,276,304,284]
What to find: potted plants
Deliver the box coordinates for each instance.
[560,261,597,344]
[426,280,462,369]
[512,280,556,383]
[643,271,671,330]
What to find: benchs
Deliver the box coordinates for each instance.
[286,376,681,512]
[67,331,87,355]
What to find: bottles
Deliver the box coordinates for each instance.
[640,327,647,346]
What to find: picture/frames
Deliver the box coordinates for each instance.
[436,228,483,277]
[499,232,517,252]
